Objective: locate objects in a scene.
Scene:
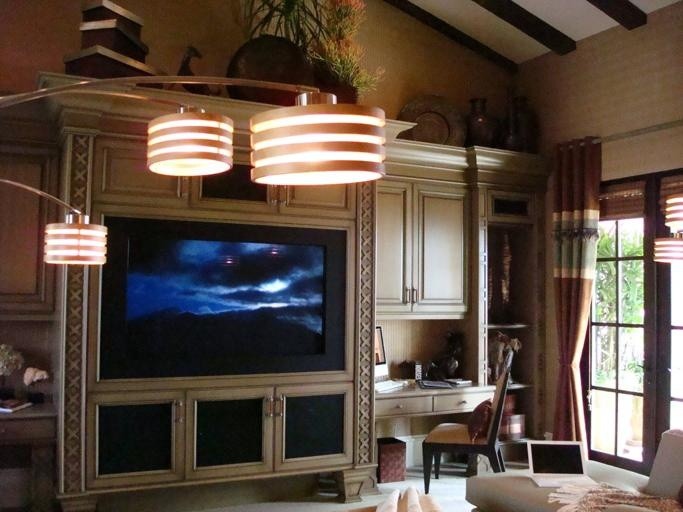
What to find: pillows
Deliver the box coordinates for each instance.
[468,398,493,440]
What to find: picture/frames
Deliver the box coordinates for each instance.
[375,327,387,365]
[394,94,467,146]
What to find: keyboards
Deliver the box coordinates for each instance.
[374,379,403,393]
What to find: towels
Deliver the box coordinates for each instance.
[375,487,441,512]
[546,479,683,512]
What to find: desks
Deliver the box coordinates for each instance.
[0,397,59,512]
[374,383,496,423]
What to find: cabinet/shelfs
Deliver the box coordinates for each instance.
[468,145,547,441]
[34,73,375,512]
[374,117,472,319]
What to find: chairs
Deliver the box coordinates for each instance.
[465,429,683,512]
[423,349,514,494]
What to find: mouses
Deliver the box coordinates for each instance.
[398,380,408,385]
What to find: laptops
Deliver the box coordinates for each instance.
[526,439,597,488]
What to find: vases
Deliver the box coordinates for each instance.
[464,95,540,152]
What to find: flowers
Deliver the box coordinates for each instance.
[0,343,25,376]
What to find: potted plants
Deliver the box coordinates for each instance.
[225,0,385,105]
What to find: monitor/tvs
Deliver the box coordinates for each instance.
[100,216,346,379]
[374,326,389,383]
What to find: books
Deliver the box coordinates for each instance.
[79,19,150,55]
[80,2,145,27]
[0,399,35,415]
[62,43,170,80]
[419,379,452,391]
[443,377,474,385]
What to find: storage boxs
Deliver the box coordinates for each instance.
[63,0,163,89]
[496,414,526,443]
